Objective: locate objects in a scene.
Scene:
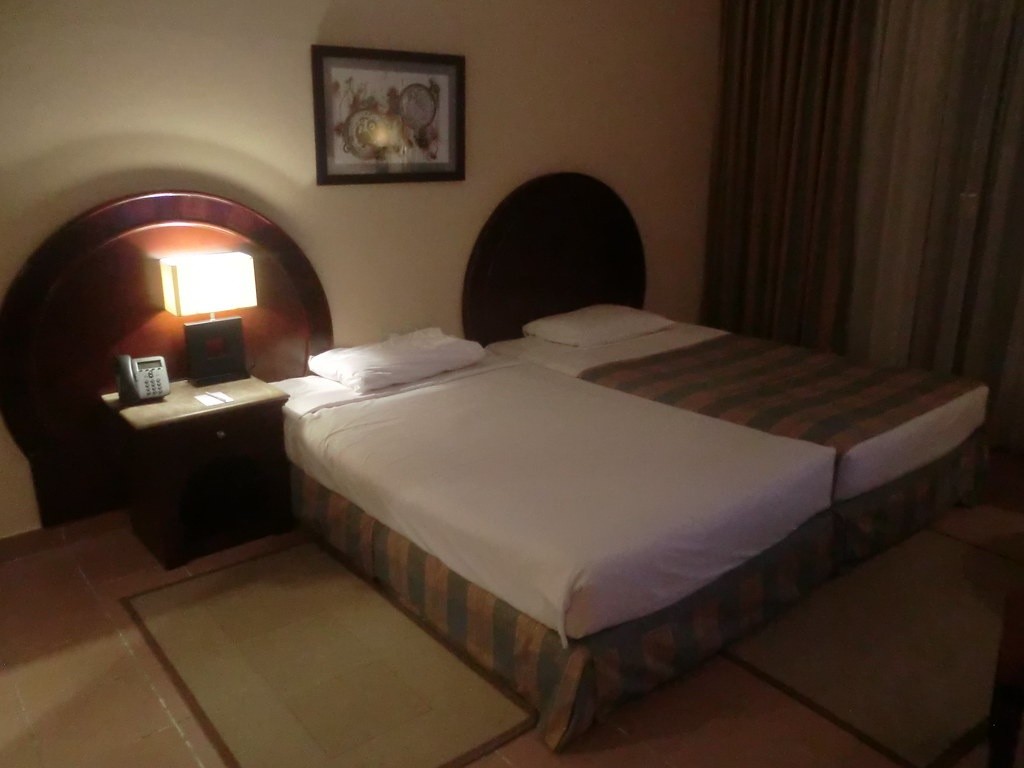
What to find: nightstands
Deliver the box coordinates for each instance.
[98,374,290,570]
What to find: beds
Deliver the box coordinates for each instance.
[267,308,989,753]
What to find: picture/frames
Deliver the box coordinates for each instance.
[310,43,465,186]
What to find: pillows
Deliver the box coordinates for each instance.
[308,326,485,395]
[521,302,677,348]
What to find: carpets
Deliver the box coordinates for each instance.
[117,540,542,768]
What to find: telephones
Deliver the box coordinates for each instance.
[117,354,171,405]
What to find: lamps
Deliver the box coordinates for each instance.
[159,252,258,385]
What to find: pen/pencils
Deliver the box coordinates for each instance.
[205,391,226,402]
